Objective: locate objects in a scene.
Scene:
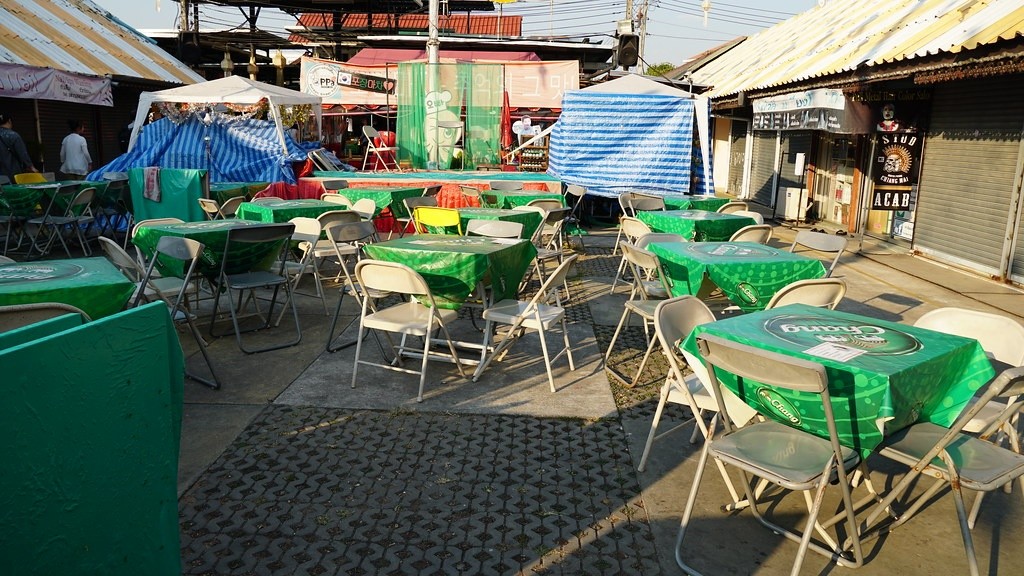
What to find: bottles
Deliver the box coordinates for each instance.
[520,148,545,172]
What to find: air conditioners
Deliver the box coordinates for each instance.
[777,187,809,220]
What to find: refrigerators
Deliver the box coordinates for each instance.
[776,186,808,220]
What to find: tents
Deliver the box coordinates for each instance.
[127,75,322,157]
[544,73,711,195]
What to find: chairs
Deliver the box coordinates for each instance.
[0,122,1024,576]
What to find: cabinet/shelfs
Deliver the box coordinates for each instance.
[518,135,549,172]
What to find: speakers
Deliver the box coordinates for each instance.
[617,34,639,66]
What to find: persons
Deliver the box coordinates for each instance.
[521,115,534,134]
[60,120,93,180]
[0,114,40,185]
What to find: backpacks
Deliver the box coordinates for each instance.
[117,126,133,153]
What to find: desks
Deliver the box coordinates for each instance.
[362,235,539,380]
[643,241,828,363]
[130,219,295,346]
[678,302,997,568]
[209,178,273,215]
[479,189,589,235]
[338,186,425,237]
[663,196,730,213]
[0,181,110,252]
[0,256,137,324]
[634,209,759,242]
[424,207,543,240]
[234,199,347,256]
